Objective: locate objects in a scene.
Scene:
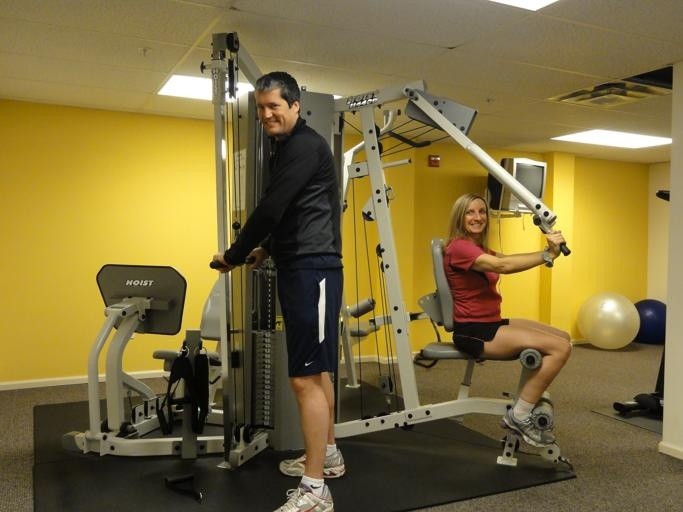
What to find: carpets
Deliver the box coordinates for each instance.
[32,379,578,512]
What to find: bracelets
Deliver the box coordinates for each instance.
[541,244,553,268]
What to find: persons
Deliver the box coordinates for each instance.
[212,70,346,512]
[440,191,567,449]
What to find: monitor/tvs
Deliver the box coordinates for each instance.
[488,156,548,215]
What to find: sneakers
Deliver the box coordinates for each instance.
[280,450,345,479]
[273,484,334,512]
[500,408,556,449]
